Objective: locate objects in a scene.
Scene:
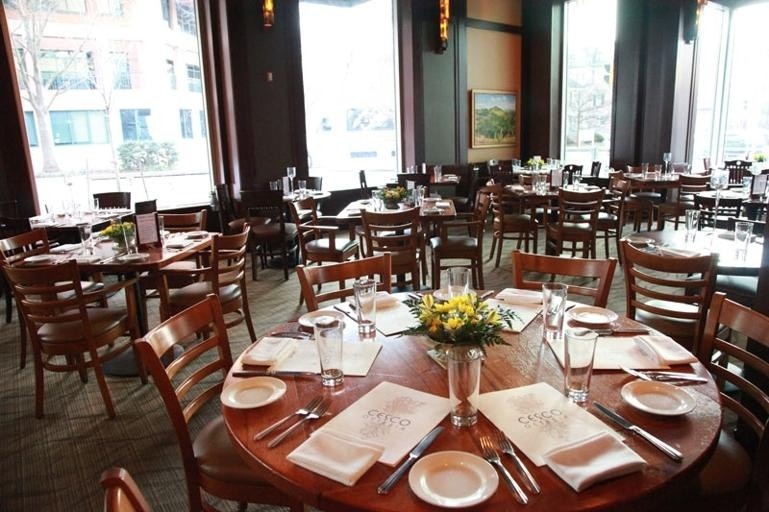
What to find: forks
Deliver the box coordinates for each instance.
[495,432,541,496]
[476,436,529,506]
[253,395,324,443]
[265,397,333,448]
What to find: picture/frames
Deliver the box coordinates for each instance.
[469,88,518,148]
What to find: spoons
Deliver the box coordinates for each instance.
[619,366,707,383]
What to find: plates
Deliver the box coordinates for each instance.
[299,310,347,331]
[24,230,209,263]
[627,235,655,245]
[411,451,499,509]
[569,305,618,324]
[434,287,476,300]
[221,375,285,409]
[621,380,696,416]
[347,197,450,216]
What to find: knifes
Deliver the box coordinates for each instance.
[592,401,685,464]
[377,427,444,496]
[592,327,649,337]
[231,371,313,379]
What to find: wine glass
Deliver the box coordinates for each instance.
[662,152,672,178]
[287,167,297,198]
[706,169,729,236]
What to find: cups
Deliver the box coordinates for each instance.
[541,281,568,336]
[314,324,347,388]
[77,223,137,256]
[434,164,442,182]
[445,346,479,427]
[564,327,598,404]
[371,189,425,212]
[448,268,469,299]
[270,178,306,200]
[44,198,100,223]
[531,170,582,196]
[685,210,754,251]
[641,163,691,179]
[353,280,376,335]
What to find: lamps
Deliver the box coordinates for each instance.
[261,0,275,28]
[439,0,450,51]
